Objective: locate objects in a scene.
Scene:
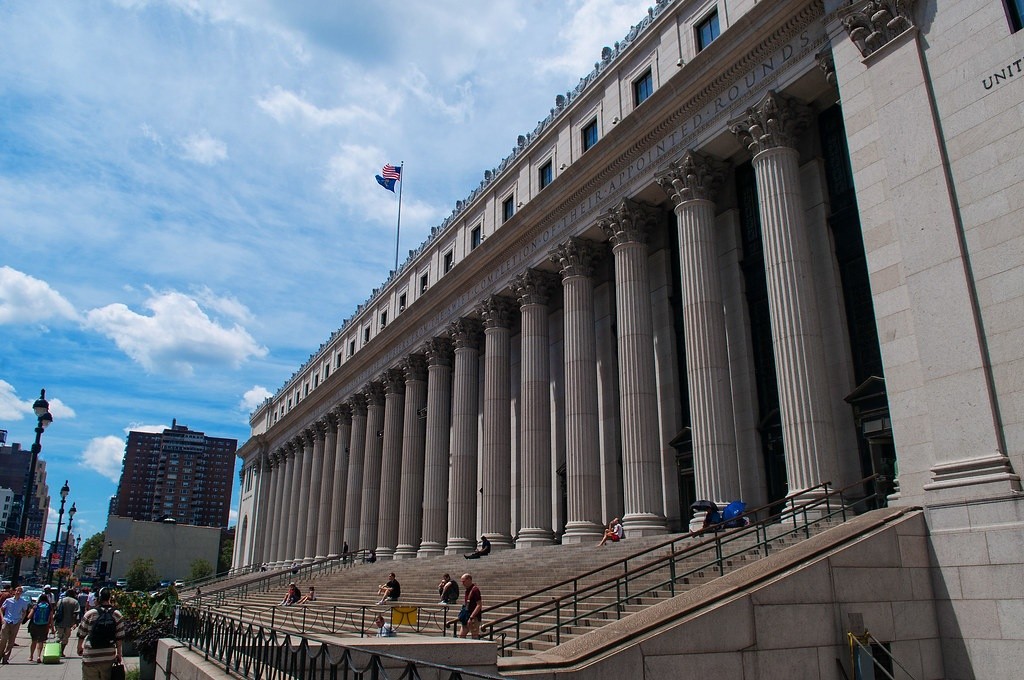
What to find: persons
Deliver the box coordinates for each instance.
[459,573,482,639]
[464,536,491,559]
[342,542,348,564]
[367,550,377,563]
[689,507,749,538]
[195,587,201,596]
[291,563,298,574]
[375,572,401,605]
[597,518,622,547]
[279,582,316,605]
[261,563,268,572]
[367,616,398,639]
[0,584,127,680]
[437,573,459,604]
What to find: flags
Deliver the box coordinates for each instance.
[375,165,401,193]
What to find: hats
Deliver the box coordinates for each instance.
[44,585,50,589]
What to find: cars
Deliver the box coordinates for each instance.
[0,580,44,603]
[116,578,127,587]
[160,579,171,587]
[174,579,185,587]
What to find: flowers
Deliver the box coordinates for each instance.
[111,589,153,635]
[53,568,72,579]
[0,535,42,559]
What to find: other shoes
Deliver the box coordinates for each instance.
[439,600,448,605]
[3,659,8,664]
[37,658,42,663]
[375,600,386,605]
[30,658,32,661]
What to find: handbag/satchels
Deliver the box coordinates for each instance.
[54,598,64,625]
[111,657,125,680]
[459,606,471,625]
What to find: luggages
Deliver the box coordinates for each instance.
[42,632,61,664]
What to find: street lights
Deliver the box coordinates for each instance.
[49,479,70,584]
[108,549,121,584]
[58,501,77,592]
[9,388,53,589]
[71,534,82,573]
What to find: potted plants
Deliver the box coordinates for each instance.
[122,618,142,657]
[137,619,173,680]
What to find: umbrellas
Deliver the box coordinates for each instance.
[722,501,748,522]
[690,500,719,512]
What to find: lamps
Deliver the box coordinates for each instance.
[560,164,568,170]
[677,59,686,67]
[423,285,430,290]
[480,234,488,240]
[450,261,457,267]
[517,201,525,207]
[612,117,621,124]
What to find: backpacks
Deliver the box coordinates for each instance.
[34,602,50,625]
[91,606,116,649]
